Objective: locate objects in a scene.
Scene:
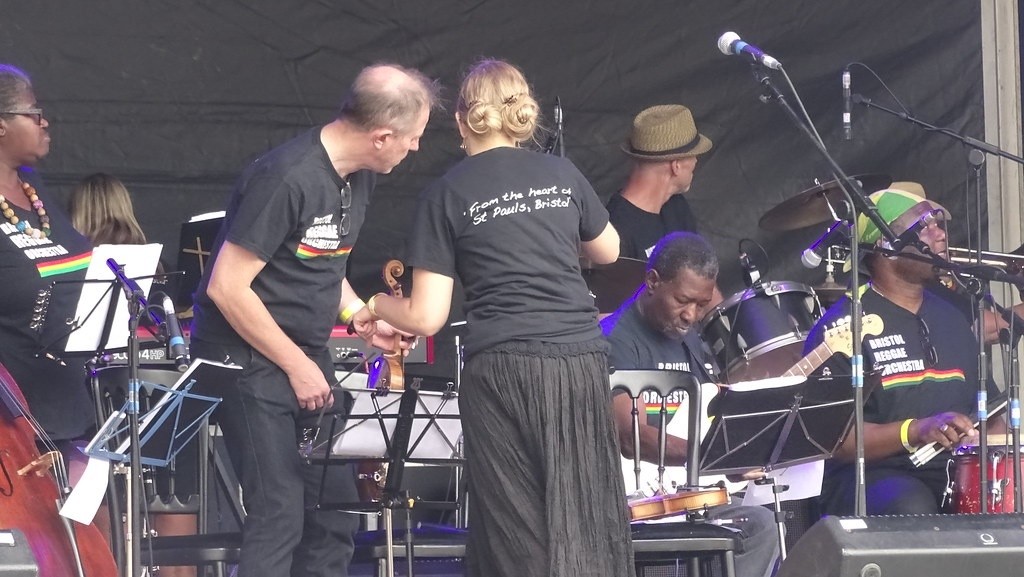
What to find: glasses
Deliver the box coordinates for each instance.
[0,108,43,126]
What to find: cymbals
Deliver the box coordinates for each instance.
[758,175,861,233]
[812,281,849,298]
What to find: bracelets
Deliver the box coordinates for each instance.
[339,298,365,323]
[900,418,919,452]
[369,292,386,314]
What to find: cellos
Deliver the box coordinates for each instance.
[0,361,119,576]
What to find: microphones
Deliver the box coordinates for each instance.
[801,218,847,268]
[884,209,936,259]
[163,295,189,373]
[740,252,753,287]
[554,103,566,158]
[842,70,852,140]
[717,31,781,71]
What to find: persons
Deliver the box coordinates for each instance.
[71,171,197,577]
[601,232,784,577]
[0,65,101,488]
[582,103,713,312]
[806,189,1024,515]
[190,64,446,577]
[354,53,635,577]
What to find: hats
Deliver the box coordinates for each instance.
[619,103,714,163]
[855,188,927,244]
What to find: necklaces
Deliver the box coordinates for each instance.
[0,175,51,237]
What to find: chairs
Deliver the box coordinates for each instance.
[90,359,468,577]
[610,366,738,577]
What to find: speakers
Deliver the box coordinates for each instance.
[775,511,1024,577]
[0,529,38,577]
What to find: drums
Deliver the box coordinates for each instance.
[940,433,1024,513]
[699,280,826,385]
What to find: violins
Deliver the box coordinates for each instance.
[356,258,407,498]
[627,481,729,523]
